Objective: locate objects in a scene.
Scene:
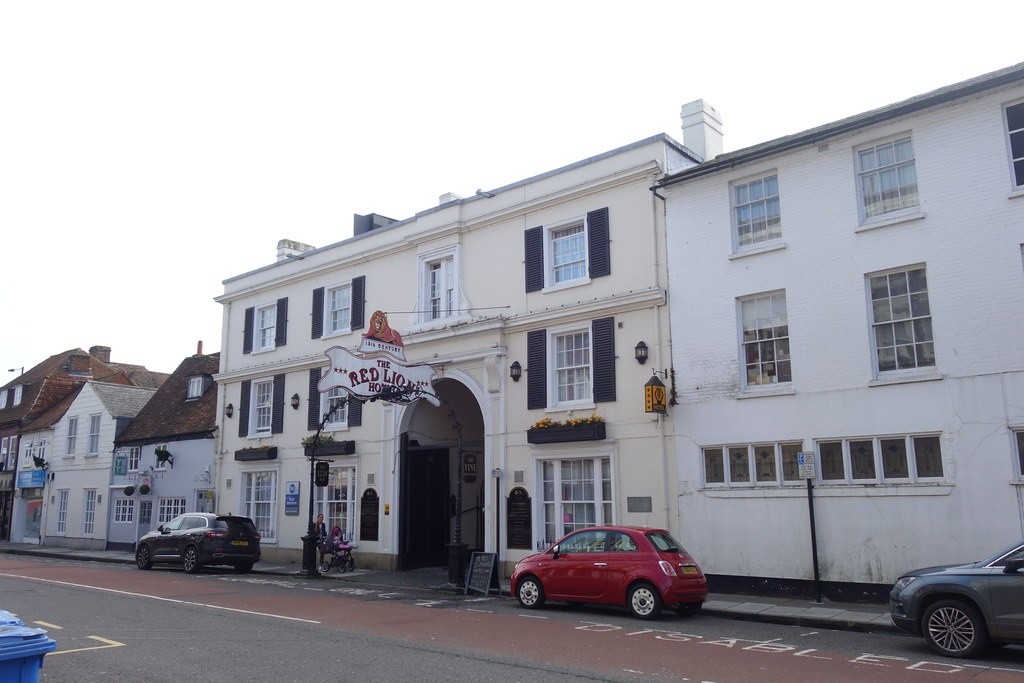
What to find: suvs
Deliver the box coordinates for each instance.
[135,513,262,573]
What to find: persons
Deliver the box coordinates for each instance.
[315,514,327,571]
[333,536,349,548]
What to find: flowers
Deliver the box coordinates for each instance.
[529,412,605,429]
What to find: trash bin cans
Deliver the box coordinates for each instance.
[0,625,56,683]
[0,609,24,625]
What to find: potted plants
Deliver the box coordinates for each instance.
[300,434,356,456]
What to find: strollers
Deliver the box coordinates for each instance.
[319,526,354,573]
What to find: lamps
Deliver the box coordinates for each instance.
[509,361,521,382]
[644,368,667,415]
[634,341,648,364]
[225,403,233,418]
[290,393,299,410]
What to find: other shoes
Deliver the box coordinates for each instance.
[319,566,322,570]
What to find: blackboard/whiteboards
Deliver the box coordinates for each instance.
[467,552,501,593]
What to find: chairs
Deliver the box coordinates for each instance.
[627,538,637,551]
[614,534,624,551]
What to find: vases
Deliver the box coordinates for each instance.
[234,447,277,461]
[527,422,606,444]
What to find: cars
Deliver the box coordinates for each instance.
[889,540,1024,658]
[510,526,708,620]
